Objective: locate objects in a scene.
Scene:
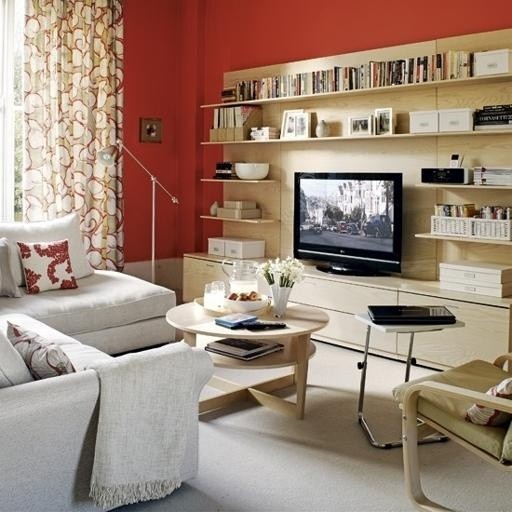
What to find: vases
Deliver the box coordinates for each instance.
[268,283,296,315]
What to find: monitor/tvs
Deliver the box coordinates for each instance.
[291,171,403,277]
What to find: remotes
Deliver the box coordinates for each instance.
[241,320,287,331]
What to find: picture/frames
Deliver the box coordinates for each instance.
[279,108,311,139]
[346,106,396,134]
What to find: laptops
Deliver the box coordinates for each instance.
[366,305,456,325]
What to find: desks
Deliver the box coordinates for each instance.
[353,313,466,451]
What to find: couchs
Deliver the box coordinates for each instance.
[0,215,210,510]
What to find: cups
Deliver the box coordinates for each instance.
[203,279,225,310]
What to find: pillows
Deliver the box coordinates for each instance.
[0,321,77,388]
[462,375,512,427]
[0,238,78,298]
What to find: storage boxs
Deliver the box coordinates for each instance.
[439,261,512,298]
[473,48,511,75]
[408,108,475,133]
[208,236,266,259]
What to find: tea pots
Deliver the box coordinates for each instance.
[219,257,260,292]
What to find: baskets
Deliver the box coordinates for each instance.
[430,216,474,237]
[473,216,512,241]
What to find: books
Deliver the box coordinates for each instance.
[215,313,257,329]
[207,338,277,356]
[205,345,285,361]
[433,203,512,220]
[213,105,256,128]
[221,49,472,104]
[473,102,512,132]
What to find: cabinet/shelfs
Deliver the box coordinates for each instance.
[415,183,512,246]
[198,70,512,145]
[200,178,281,225]
[182,253,512,371]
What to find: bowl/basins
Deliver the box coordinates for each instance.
[234,160,270,181]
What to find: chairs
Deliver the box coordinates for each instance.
[391,353,512,512]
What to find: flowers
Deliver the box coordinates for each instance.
[256,255,307,287]
